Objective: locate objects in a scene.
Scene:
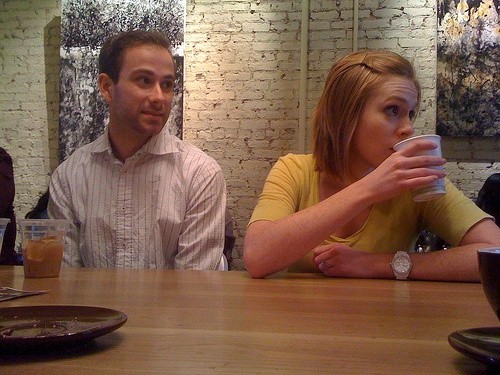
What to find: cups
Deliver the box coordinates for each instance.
[478,247,500,319]
[393,134,446,203]
[17,220,70,278]
[0,219,10,252]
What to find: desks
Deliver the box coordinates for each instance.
[0,263,500,375]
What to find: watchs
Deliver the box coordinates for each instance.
[390,251,412,280]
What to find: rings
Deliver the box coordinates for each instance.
[324,260,330,268]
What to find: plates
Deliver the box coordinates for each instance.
[0,305,127,352]
[448,326,500,369]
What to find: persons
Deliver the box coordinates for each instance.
[0,146,48,265]
[46,30,236,270]
[476,173,500,227]
[243,50,500,282]
